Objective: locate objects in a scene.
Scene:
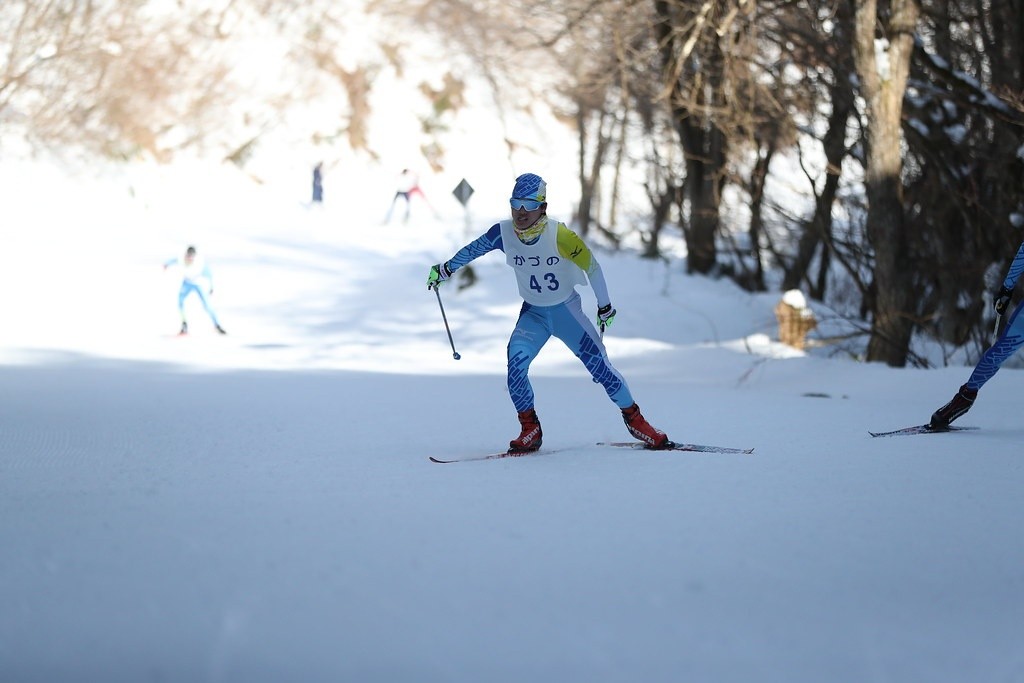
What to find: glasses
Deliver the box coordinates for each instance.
[510,198,541,212]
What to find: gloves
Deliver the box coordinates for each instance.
[994,285,1014,317]
[597,303,617,332]
[426,262,451,290]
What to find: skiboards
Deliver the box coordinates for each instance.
[428,439,755,464]
[867,423,981,438]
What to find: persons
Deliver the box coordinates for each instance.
[165,247,226,335]
[312,161,323,203]
[383,169,415,225]
[932,242,1024,429]
[426,173,667,451]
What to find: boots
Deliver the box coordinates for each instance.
[510,408,542,452]
[930,384,978,429]
[620,402,668,449]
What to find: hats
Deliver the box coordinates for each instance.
[512,173,547,203]
